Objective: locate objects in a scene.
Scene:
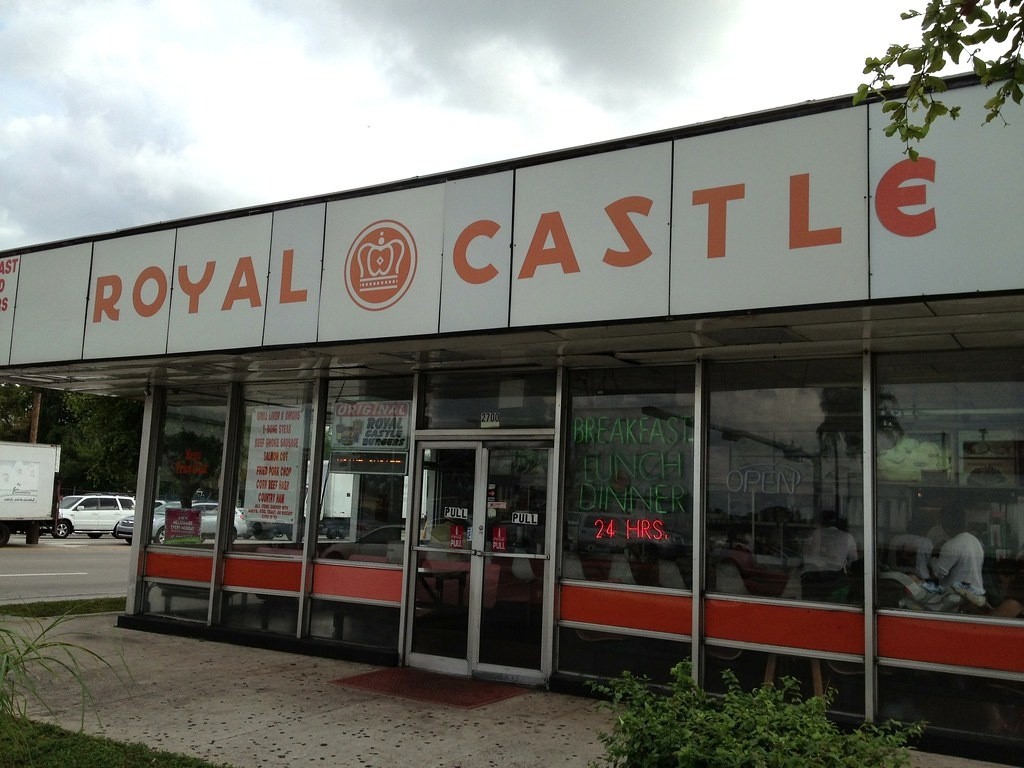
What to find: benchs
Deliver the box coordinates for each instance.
[157,582,237,623]
[417,559,500,610]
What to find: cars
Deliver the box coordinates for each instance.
[113,500,248,546]
[245,505,388,546]
[320,522,470,569]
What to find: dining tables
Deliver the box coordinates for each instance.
[415,566,469,623]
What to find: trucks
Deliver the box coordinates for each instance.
[0,440,61,546]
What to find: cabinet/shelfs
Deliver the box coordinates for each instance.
[706,477,863,556]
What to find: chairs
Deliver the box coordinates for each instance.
[512,548,865,708]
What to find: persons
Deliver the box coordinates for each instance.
[987,574,1024,618]
[802,511,858,597]
[426,518,473,561]
[888,507,986,606]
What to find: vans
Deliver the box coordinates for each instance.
[51,492,139,540]
